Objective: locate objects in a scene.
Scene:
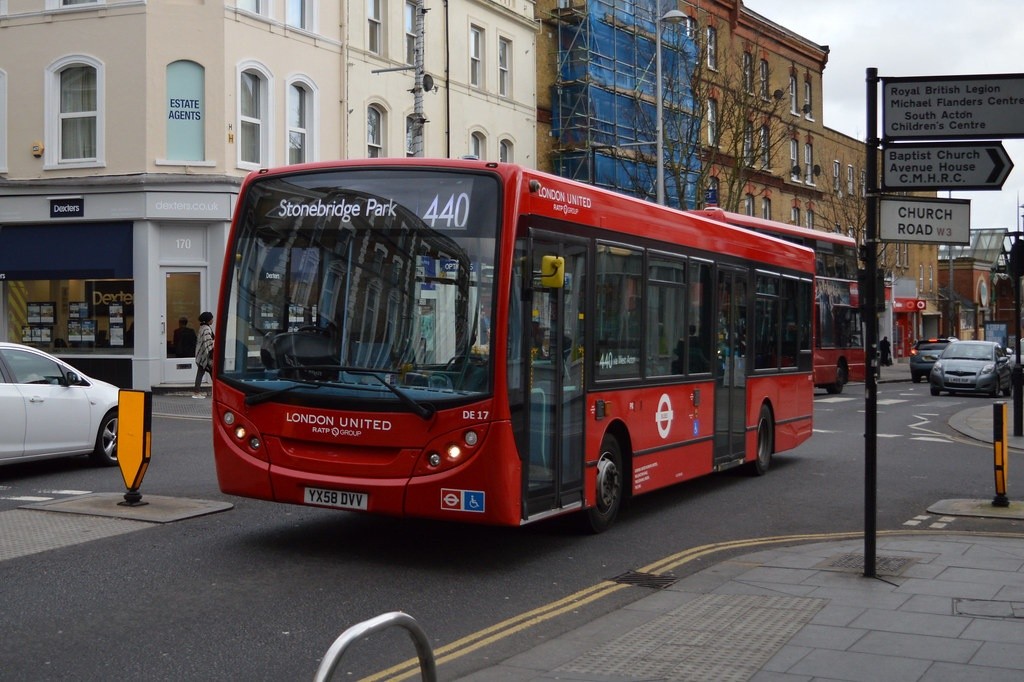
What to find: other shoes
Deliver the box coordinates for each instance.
[192,393,205,399]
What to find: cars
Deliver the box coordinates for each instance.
[911,336,960,383]
[0,342,120,468]
[929,340,1012,397]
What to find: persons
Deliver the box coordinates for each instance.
[173,317,197,358]
[192,311,215,398]
[879,337,892,367]
[535,328,550,360]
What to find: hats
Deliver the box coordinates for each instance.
[198,312,213,322]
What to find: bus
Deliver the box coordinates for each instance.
[686,206,863,394]
[207,156,814,537]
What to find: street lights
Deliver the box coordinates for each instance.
[656,8,687,205]
